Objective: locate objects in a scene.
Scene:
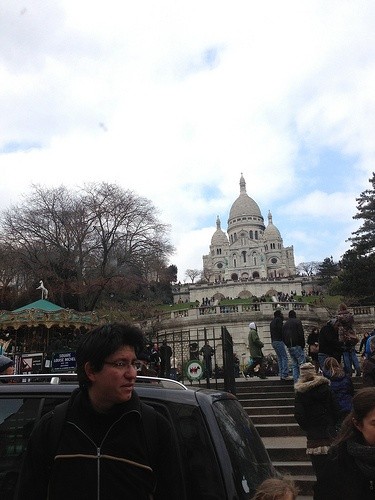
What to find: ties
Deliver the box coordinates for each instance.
[1,346,2,353]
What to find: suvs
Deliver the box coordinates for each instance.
[0,372,289,500]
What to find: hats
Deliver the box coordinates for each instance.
[300,362,316,378]
[0,355,14,373]
[249,321,256,330]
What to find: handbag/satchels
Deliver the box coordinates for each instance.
[310,343,319,353]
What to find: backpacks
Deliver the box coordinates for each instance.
[342,321,359,347]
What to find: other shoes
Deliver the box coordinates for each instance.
[356,368,362,376]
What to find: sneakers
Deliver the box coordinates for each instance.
[262,376,268,379]
[282,376,290,381]
[242,371,247,379]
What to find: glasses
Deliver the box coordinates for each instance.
[100,360,144,372]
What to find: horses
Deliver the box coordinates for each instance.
[36,280,48,299]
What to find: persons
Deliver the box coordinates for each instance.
[313,387,375,500]
[322,357,355,418]
[294,362,340,481]
[0,274,375,387]
[253,478,298,500]
[15,323,185,500]
